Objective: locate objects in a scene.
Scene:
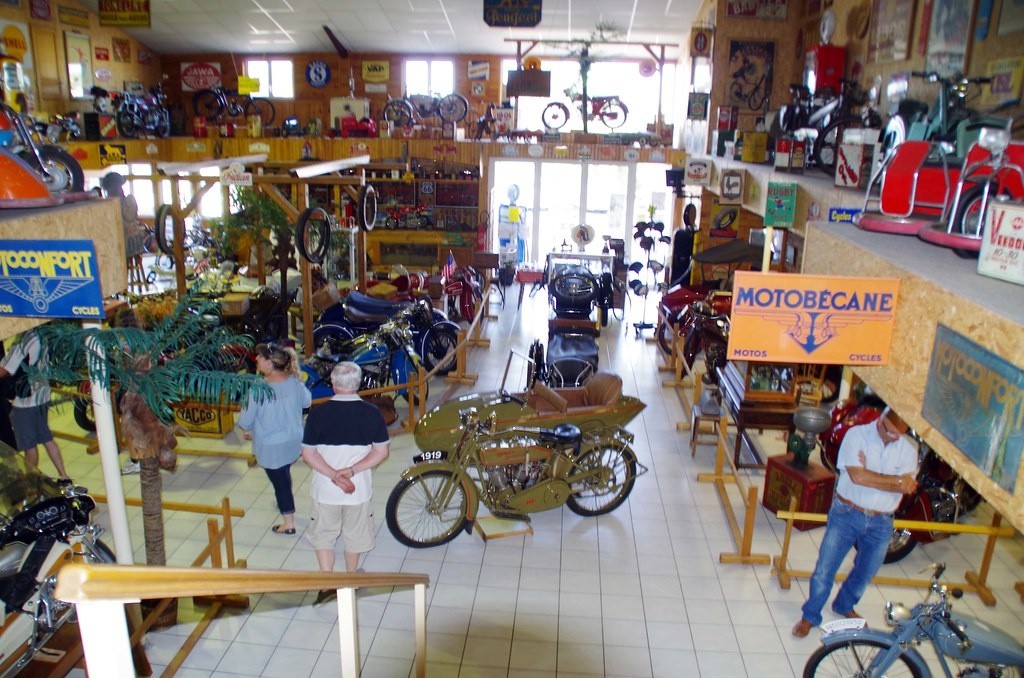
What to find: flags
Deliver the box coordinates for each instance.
[440,253,457,281]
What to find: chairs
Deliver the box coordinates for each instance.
[583,369,623,407]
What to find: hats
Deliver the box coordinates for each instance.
[883,404,908,433]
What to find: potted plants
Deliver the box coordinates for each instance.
[19,283,268,631]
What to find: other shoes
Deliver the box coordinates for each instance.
[271,525,296,535]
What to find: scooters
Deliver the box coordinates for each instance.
[382,91,468,126]
[190,82,276,127]
[765,67,1024,262]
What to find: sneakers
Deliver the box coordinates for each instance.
[121,460,140,475]
[313,589,336,608]
[352,568,364,590]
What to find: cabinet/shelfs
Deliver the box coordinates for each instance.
[252,157,489,271]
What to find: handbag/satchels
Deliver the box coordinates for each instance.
[13,368,32,398]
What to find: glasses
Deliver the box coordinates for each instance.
[881,422,904,441]
[266,342,273,355]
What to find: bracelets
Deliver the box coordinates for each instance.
[896,474,903,493]
[348,467,354,477]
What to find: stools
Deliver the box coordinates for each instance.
[690,405,727,459]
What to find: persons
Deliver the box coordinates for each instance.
[120,456,141,475]
[0,326,74,487]
[301,362,391,610]
[791,407,920,638]
[237,342,312,535]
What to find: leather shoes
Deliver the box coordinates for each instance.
[834,607,860,618]
[792,618,813,636]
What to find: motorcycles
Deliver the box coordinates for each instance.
[541,84,629,130]
[1,441,115,677]
[309,263,483,409]
[0,93,84,195]
[385,345,650,549]
[115,82,171,141]
[818,377,983,566]
[72,280,294,433]
[803,562,1024,678]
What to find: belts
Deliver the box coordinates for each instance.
[836,493,888,517]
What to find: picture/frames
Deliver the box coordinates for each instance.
[726,0,790,20]
[924,0,976,82]
[64,31,95,98]
[726,37,777,115]
[867,0,917,65]
